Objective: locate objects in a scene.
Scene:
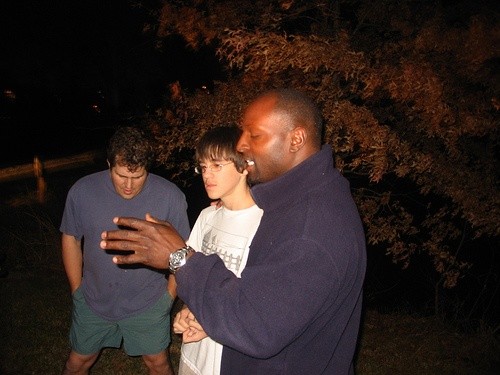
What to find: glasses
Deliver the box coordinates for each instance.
[195,162,233,174]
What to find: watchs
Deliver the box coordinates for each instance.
[168,246,196,275]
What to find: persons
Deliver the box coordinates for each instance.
[173,125,263,375]
[60,126,190,375]
[100,90,368,375]
